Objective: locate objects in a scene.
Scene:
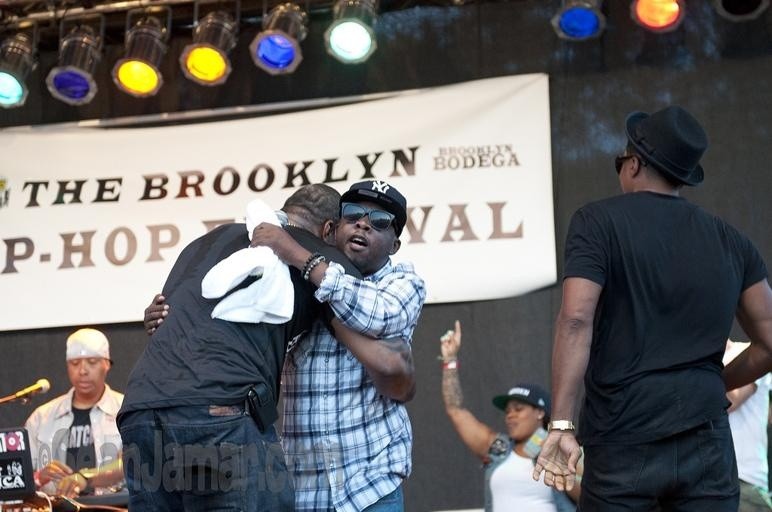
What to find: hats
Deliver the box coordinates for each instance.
[624,105,708,187]
[491,382,552,424]
[65,328,115,365]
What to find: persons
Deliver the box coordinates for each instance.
[21,325,129,495]
[530,103,771,511]
[433,319,578,512]
[717,334,772,511]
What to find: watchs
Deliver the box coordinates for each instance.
[78,466,95,493]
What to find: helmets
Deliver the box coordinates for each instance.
[338,179,408,237]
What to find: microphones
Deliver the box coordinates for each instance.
[14,379,51,398]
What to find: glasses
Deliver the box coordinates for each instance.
[339,202,400,236]
[615,155,647,175]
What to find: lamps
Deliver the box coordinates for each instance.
[552,1,772,46]
[0,0,382,114]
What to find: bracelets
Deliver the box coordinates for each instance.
[32,469,47,488]
[543,418,577,433]
[437,356,460,370]
[297,250,320,278]
[304,253,325,281]
